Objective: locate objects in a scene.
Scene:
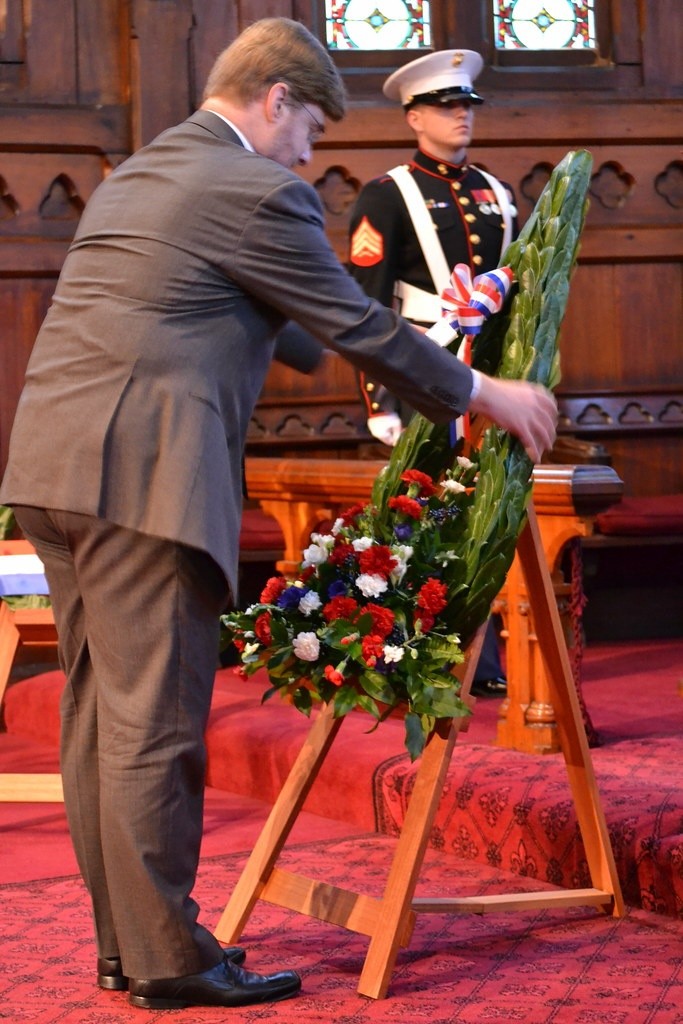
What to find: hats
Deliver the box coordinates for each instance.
[382,49,485,111]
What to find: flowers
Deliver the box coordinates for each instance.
[216,462,475,765]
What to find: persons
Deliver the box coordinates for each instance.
[350,50,519,697]
[0,16,560,1006]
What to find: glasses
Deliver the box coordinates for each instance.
[269,78,326,140]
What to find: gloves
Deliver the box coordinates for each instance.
[366,412,401,448]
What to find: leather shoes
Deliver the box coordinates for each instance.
[95,946,246,990]
[128,955,302,1010]
[470,675,510,698]
